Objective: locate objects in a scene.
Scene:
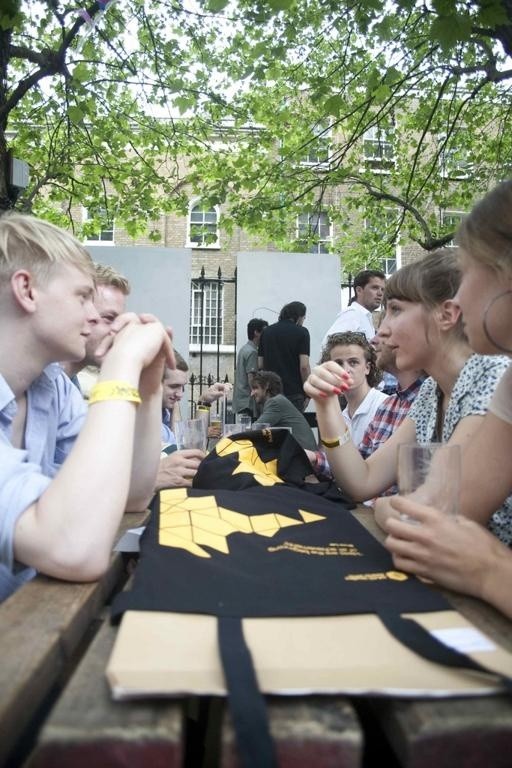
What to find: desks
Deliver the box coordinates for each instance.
[1,474,511,768]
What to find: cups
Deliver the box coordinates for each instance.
[210,413,271,437]
[396,442,464,586]
[174,417,207,456]
